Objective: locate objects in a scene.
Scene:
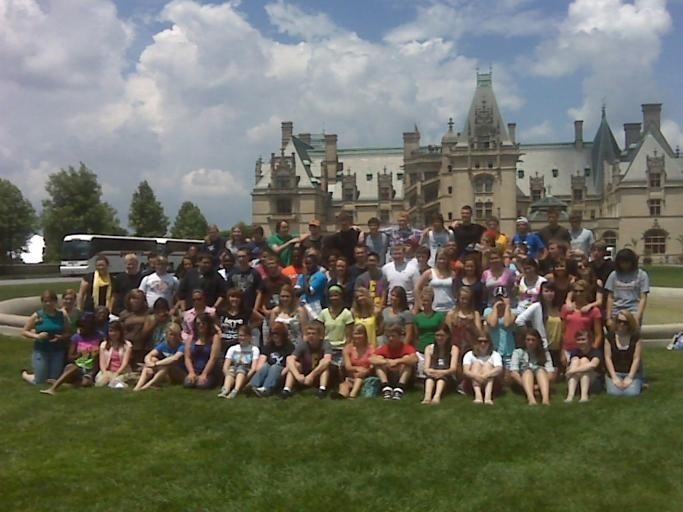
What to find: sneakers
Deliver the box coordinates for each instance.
[382,387,404,400]
[281,388,292,398]
[316,387,327,400]
[217,387,238,399]
[244,383,272,397]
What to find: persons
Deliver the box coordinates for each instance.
[18,202,649,411]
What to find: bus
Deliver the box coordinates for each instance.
[59,235,209,276]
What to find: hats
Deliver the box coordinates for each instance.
[494,287,507,296]
[309,220,320,226]
[517,216,528,223]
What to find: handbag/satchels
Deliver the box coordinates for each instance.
[362,376,379,397]
